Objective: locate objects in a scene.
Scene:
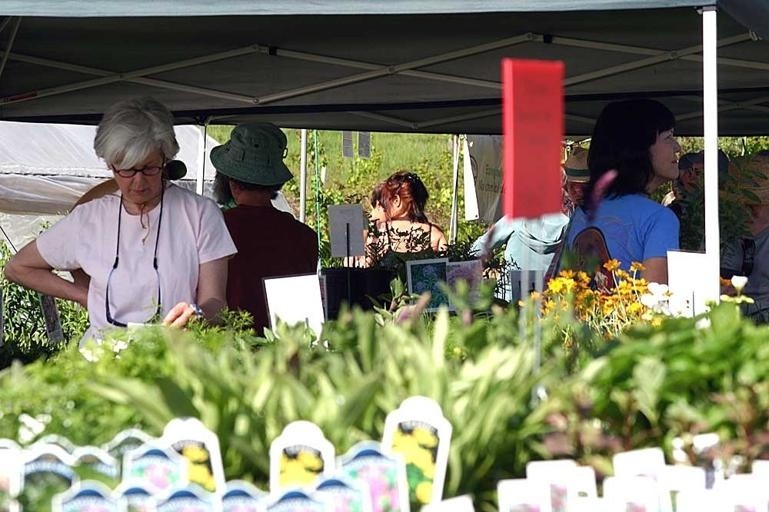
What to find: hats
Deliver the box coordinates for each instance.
[209,122,293,187]
[685,149,729,172]
[563,147,592,186]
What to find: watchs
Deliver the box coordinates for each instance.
[187,300,206,324]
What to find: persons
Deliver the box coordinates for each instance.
[65,159,190,289]
[471,165,570,301]
[720,149,769,324]
[193,123,322,338]
[670,150,695,227]
[559,98,682,287]
[362,169,446,270]
[561,146,591,209]
[680,149,729,253]
[342,181,390,272]
[3,96,240,350]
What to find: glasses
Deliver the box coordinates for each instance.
[111,152,166,178]
